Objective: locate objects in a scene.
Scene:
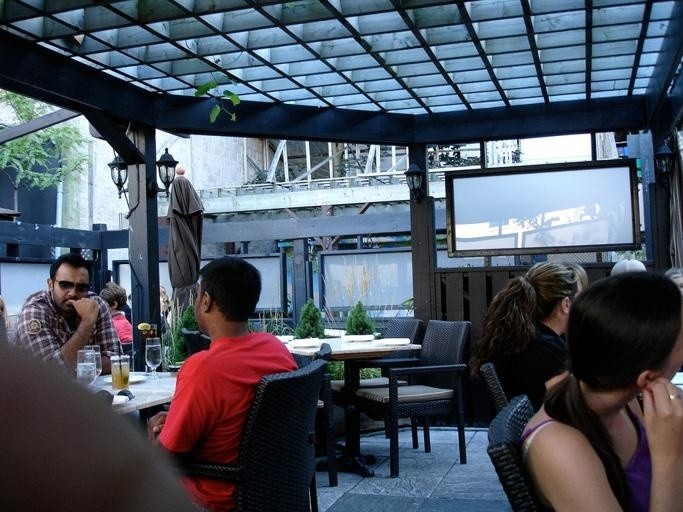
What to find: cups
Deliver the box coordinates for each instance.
[82,345,100,363]
[144,337,162,386]
[109,355,130,391]
[84,353,102,385]
[77,349,96,389]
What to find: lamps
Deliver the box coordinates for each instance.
[654,140,676,178]
[153,146,180,199]
[107,152,128,201]
[402,161,426,202]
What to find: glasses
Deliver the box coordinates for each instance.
[53,279,92,292]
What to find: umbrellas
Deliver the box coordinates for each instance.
[166,164,205,337]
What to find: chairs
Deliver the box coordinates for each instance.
[486,392,554,512]
[354,319,473,479]
[477,360,512,410]
[172,357,331,512]
[331,315,424,450]
[310,342,339,508]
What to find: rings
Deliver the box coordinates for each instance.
[668,394,681,401]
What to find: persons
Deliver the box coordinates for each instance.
[609,258,647,273]
[469,260,588,412]
[666,266,683,295]
[518,269,683,512]
[0,296,199,512]
[145,254,298,511]
[160,285,172,318]
[9,250,127,378]
[98,277,137,345]
